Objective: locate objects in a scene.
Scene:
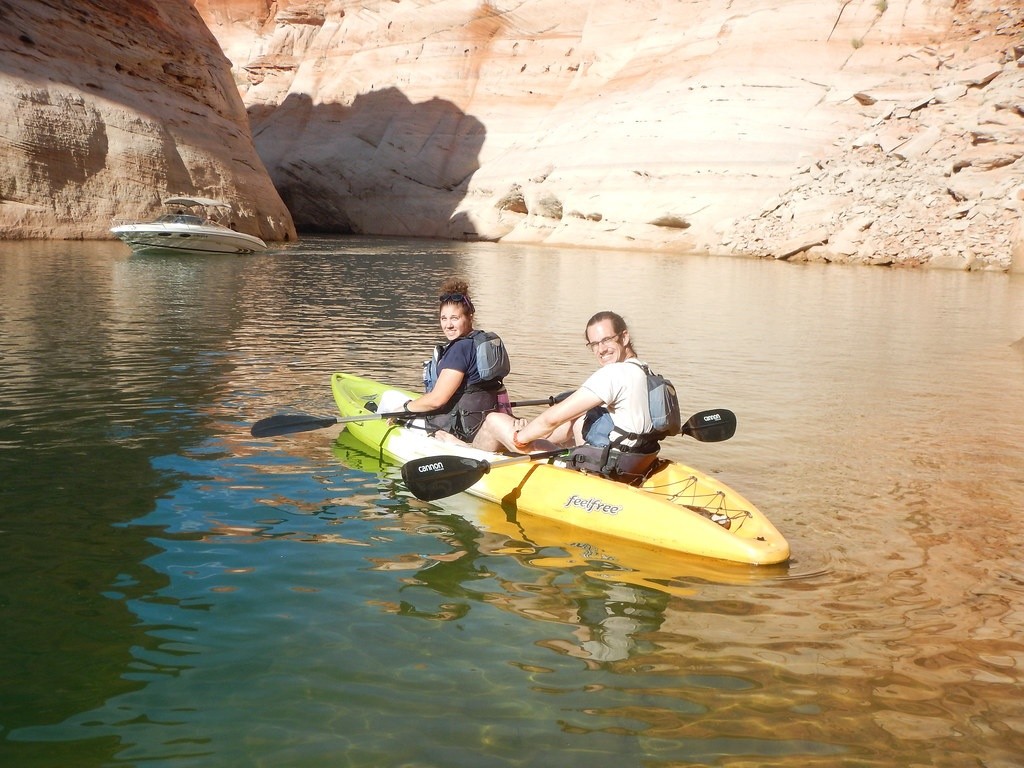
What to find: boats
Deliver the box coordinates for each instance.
[328,372,791,567]
[108,195,269,256]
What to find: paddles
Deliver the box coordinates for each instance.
[251,386,578,441]
[403,407,736,502]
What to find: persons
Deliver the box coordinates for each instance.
[433,310,657,473]
[364,277,499,444]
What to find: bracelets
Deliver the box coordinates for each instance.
[404,399,413,414]
[513,430,528,448]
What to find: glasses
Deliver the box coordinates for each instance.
[586,332,622,352]
[440,294,470,308]
